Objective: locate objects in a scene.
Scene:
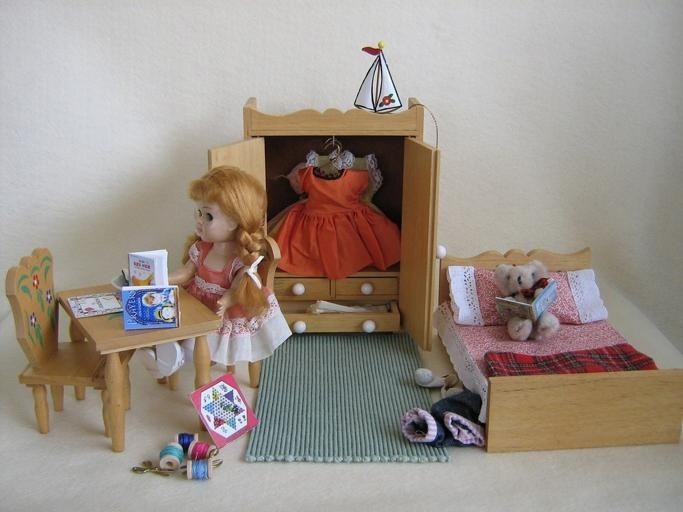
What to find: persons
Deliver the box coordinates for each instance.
[142,165,293,381]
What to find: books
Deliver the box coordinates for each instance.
[67,290,122,319]
[494,279,557,322]
[126,248,169,286]
[121,285,180,330]
